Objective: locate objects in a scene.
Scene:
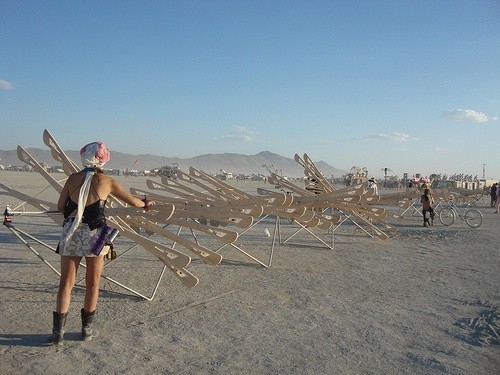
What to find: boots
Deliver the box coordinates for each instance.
[49,310,69,345]
[81,307,96,341]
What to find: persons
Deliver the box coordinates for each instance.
[490,182,500,214]
[3,205,14,224]
[48,142,156,345]
[409,180,428,203]
[306,169,323,195]
[421,186,436,228]
[366,177,380,202]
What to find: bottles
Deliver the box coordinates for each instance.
[100,229,120,256]
[91,226,113,256]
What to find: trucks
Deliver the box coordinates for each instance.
[221,173,233,180]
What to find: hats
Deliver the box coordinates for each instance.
[80,141,110,168]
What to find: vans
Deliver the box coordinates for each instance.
[51,166,64,173]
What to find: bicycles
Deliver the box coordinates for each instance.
[438,196,483,228]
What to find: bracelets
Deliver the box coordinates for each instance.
[143,199,149,209]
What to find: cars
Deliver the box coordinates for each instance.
[129,170,141,176]
[11,164,22,171]
